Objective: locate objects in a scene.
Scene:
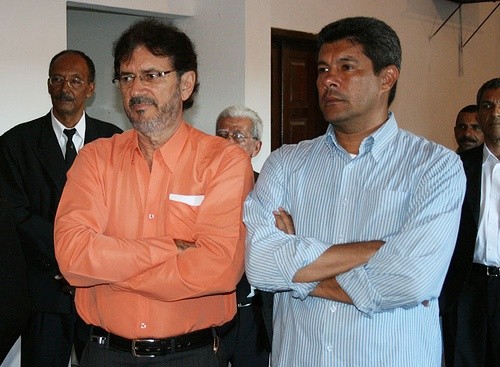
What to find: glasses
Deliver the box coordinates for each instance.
[48,75,89,88]
[111,68,176,90]
[217,130,260,143]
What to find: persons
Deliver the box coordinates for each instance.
[439,77,500,367]
[243,18,466,367]
[209,105,275,367]
[0,51,124,367]
[55,20,254,367]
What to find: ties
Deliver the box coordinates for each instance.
[63,128,78,170]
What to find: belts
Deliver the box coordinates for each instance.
[472,263,500,277]
[236,302,258,313]
[88,312,239,358]
